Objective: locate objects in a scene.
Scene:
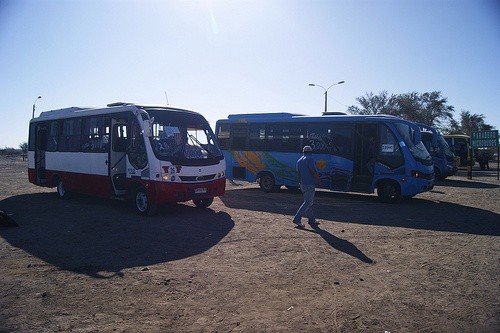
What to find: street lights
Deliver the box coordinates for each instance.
[309,80,345,111]
[32,96,41,118]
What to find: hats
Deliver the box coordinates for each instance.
[303,146,312,152]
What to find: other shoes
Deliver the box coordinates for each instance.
[308,219,320,223]
[293,220,305,227]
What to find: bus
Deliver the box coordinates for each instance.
[26,102,227,215]
[415,122,456,182]
[442,133,474,172]
[214,113,436,205]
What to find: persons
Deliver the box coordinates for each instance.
[292,146,321,226]
